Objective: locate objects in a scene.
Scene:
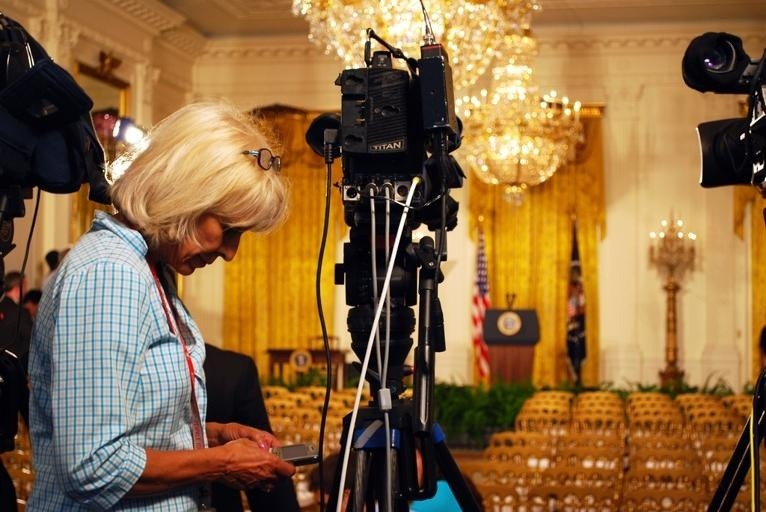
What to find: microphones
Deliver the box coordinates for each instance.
[505,291,510,304]
[511,292,516,305]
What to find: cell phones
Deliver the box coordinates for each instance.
[268,444,319,466]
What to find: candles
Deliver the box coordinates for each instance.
[648,219,698,259]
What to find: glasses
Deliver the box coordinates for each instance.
[244,147,283,172]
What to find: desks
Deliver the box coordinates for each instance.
[267,346,348,391]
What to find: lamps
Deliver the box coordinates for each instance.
[456,1,581,206]
[291,2,518,99]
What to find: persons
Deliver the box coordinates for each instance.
[22,99,296,509]
[307,448,358,511]
[0,246,72,511]
[202,341,301,512]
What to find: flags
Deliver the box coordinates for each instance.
[469,226,491,378]
[565,223,587,387]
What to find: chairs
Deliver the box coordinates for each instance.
[309,334,339,388]
[477,393,757,510]
[259,379,373,458]
